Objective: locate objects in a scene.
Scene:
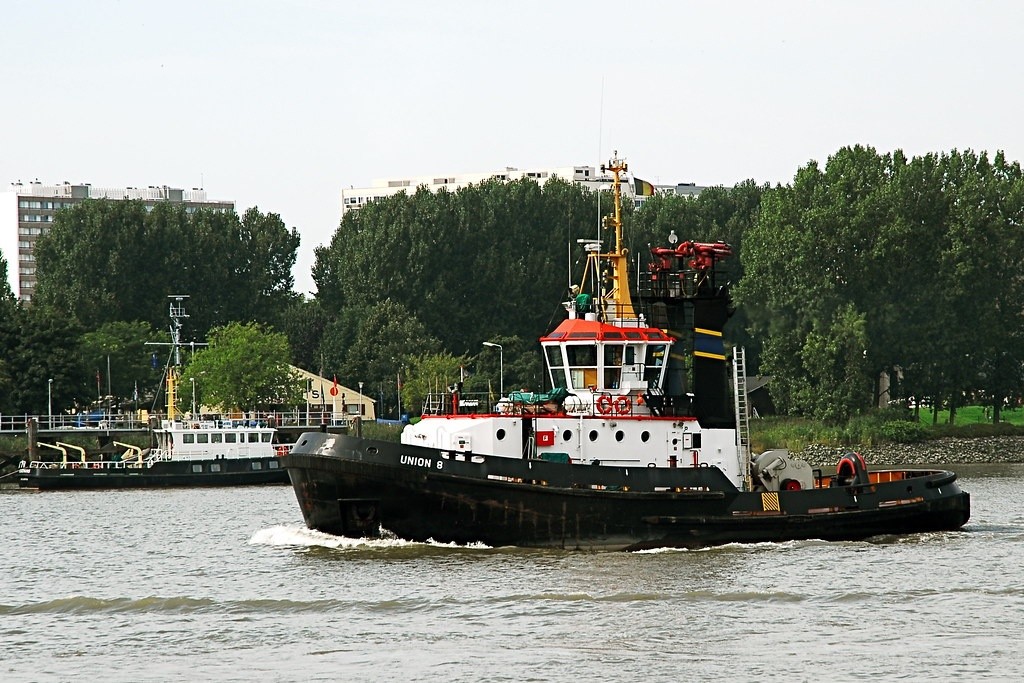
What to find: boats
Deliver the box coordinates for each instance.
[17,295,292,491]
[286,149,971,555]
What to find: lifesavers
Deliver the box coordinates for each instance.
[615,396,632,415]
[596,395,613,415]
[278,445,289,456]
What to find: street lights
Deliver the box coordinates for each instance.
[482,341,503,398]
[48,379,53,430]
